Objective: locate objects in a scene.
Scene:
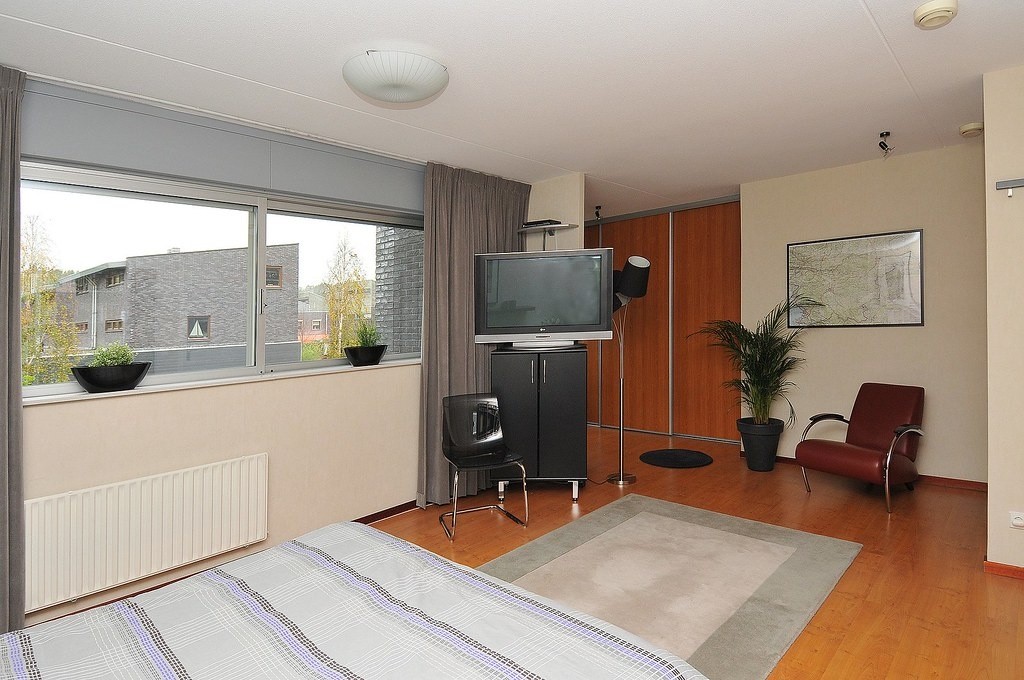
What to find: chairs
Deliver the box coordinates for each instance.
[438,392,529,542]
[795,383,925,513]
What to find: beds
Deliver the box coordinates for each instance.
[0,518,715,679]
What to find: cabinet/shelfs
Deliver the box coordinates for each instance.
[490,343,589,501]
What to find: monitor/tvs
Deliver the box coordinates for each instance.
[474,248,613,351]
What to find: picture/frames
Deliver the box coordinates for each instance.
[786,228,925,329]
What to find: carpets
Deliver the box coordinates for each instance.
[474,492,864,680]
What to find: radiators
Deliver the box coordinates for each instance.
[23,451,271,617]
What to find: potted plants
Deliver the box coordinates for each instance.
[683,290,826,472]
[343,320,389,367]
[70,339,153,393]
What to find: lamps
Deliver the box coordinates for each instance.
[586,254,651,487]
[342,50,449,104]
